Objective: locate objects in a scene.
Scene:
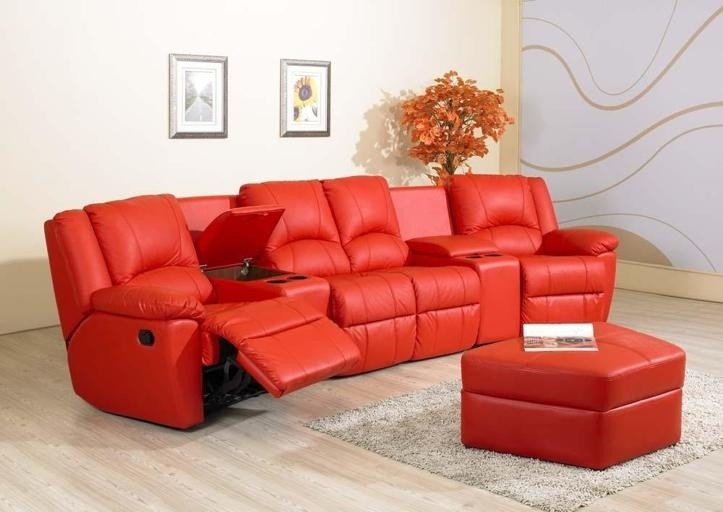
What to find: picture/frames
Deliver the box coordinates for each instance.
[280,58,331,138]
[168,52,228,139]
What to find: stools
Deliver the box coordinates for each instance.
[460,321,686,472]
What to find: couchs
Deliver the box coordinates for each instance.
[321,174,620,361]
[45,178,415,431]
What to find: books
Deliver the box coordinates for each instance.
[522,323,599,353]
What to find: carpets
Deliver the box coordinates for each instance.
[303,365,723,512]
